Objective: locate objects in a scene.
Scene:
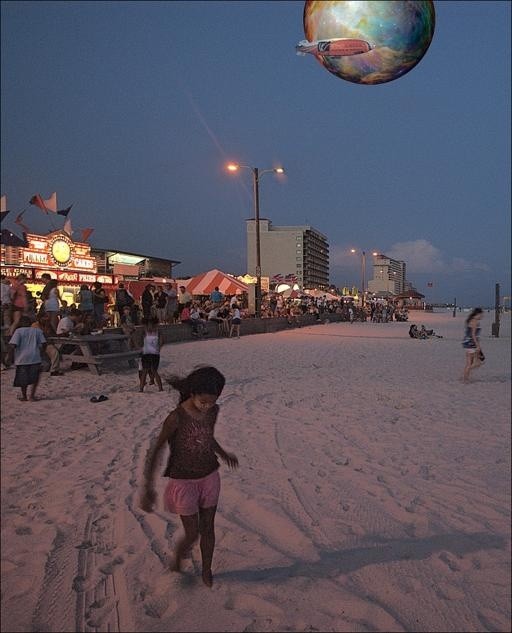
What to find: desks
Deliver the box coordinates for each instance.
[41,334,143,376]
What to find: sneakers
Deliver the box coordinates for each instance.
[158,387,164,392]
[148,381,154,386]
[139,388,143,392]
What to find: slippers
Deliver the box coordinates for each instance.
[97,394,108,402]
[90,396,98,403]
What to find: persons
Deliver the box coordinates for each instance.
[369,302,409,322]
[120,306,136,334]
[137,367,239,588]
[58,300,71,316]
[261,296,288,317]
[28,289,43,322]
[209,286,224,308]
[152,286,157,300]
[9,316,48,401]
[287,297,317,318]
[56,309,84,338]
[138,350,155,385]
[142,284,153,323]
[462,308,485,383]
[323,295,328,308]
[155,286,169,325]
[224,294,244,303]
[228,303,241,339]
[208,308,228,337]
[347,306,354,324]
[328,297,343,312]
[317,297,322,308]
[0,275,8,329]
[182,303,194,327]
[6,279,14,323]
[196,299,208,310]
[189,303,205,330]
[138,314,164,393]
[177,286,192,324]
[163,283,176,325]
[92,282,105,328]
[115,283,135,318]
[40,274,59,335]
[69,303,81,314]
[8,274,28,336]
[408,324,429,339]
[51,279,62,305]
[78,284,93,313]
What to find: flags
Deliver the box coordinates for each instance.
[59,199,76,220]
[284,273,297,283]
[270,273,281,283]
[12,208,28,233]
[62,216,74,237]
[80,227,93,243]
[29,192,54,231]
[43,191,60,230]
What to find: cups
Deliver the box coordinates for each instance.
[92,332,99,336]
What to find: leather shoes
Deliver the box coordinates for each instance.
[50,371,64,377]
[70,364,81,370]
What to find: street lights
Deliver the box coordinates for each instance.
[351,249,379,307]
[227,161,285,317]
